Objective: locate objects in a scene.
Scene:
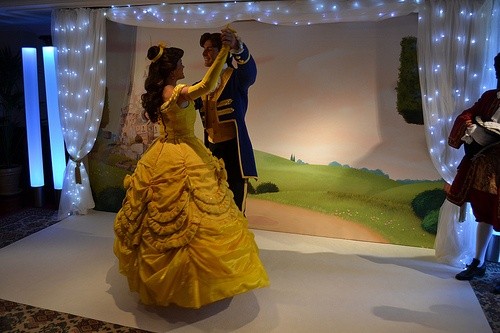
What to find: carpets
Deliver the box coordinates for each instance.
[1,298,155,333]
[0,206,64,250]
[468,260,500,333]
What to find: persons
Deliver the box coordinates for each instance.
[189,28,260,220]
[447,51,500,282]
[113,22,269,308]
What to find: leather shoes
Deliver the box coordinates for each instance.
[456,258,486,280]
[493,281,500,293]
